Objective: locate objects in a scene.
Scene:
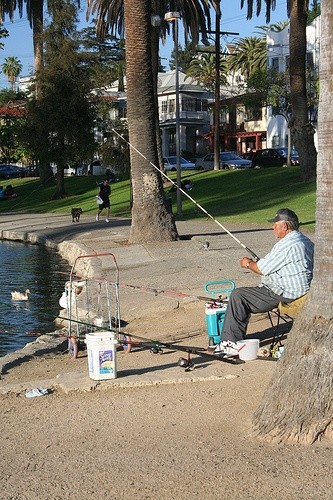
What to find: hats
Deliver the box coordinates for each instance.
[268,208,298,222]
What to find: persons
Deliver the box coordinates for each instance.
[96,178,111,223]
[0,185,17,200]
[208,208,314,354]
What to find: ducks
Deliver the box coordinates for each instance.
[11,289,30,300]
[59,280,83,309]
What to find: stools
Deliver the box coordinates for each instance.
[267,293,309,354]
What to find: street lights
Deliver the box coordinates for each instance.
[164,11,180,216]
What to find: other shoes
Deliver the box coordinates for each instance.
[96,215,99,221]
[105,218,110,222]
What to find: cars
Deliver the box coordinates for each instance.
[162,156,196,171]
[237,152,257,161]
[87,159,119,175]
[0,164,26,179]
[194,153,251,170]
[251,148,300,168]
[55,162,85,176]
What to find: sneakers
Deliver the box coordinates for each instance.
[213,341,239,358]
[207,341,228,354]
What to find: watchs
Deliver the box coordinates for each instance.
[247,260,252,269]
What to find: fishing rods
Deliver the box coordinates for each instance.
[109,126,260,262]
[56,270,229,308]
[20,306,236,372]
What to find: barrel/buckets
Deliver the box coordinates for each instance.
[85,331,117,381]
[236,339,259,361]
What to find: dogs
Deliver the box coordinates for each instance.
[71,208,82,222]
